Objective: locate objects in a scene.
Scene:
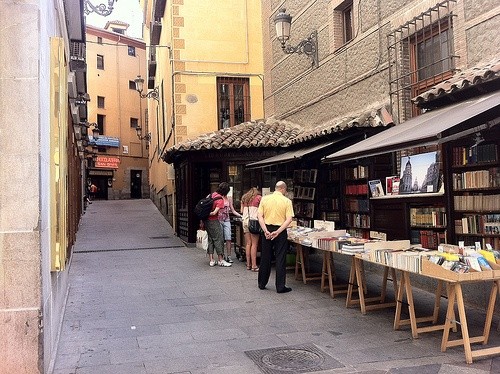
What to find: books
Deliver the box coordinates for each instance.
[263,171,277,192]
[286,143,500,274]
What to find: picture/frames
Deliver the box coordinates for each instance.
[309,169,317,183]
[293,186,301,198]
[298,187,304,198]
[308,188,315,200]
[303,187,310,198]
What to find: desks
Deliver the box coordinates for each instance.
[285,225,500,364]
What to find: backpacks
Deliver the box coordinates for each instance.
[195,194,222,221]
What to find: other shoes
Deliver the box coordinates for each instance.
[223,256,225,260]
[247,265,251,270]
[251,268,259,272]
[260,287,265,289]
[227,256,233,263]
[277,288,292,293]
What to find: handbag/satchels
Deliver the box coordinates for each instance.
[248,220,262,234]
[196,230,208,251]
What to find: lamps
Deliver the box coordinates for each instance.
[79,143,98,159]
[273,7,319,69]
[73,121,100,142]
[134,74,160,102]
[135,126,152,145]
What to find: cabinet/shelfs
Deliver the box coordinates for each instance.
[311,126,500,251]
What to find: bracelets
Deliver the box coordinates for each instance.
[240,214,242,218]
[264,230,268,233]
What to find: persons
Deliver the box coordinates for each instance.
[89,183,98,201]
[256,181,292,294]
[200,182,243,267]
[240,183,262,272]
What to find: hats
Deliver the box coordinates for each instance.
[218,182,230,194]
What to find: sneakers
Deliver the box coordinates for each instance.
[218,259,232,267]
[210,260,218,266]
